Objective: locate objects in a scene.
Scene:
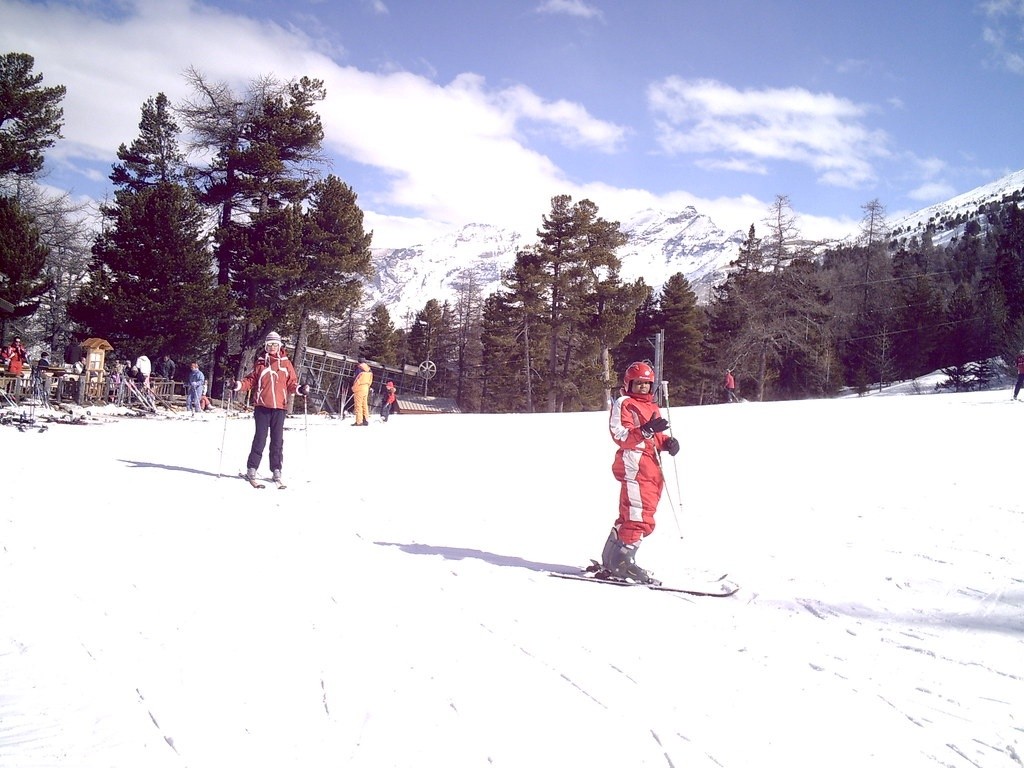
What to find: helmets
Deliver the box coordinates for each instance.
[624,362,655,394]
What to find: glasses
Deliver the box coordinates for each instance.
[267,343,279,346]
[15,341,20,343]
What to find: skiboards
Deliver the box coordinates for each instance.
[546,571,740,598]
[238,472,287,490]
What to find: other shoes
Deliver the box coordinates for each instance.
[351,421,368,426]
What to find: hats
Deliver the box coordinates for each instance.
[264,331,281,365]
[41,352,49,358]
[358,356,365,363]
[387,382,393,388]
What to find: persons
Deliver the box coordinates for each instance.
[1012,349,1024,399]
[600,361,679,585]
[350,362,373,426]
[379,381,396,421]
[724,369,734,403]
[0,336,216,416]
[224,331,310,480]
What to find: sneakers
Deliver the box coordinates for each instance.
[272,469,281,480]
[246,468,256,479]
[602,527,649,582]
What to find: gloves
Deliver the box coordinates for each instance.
[640,412,671,436]
[298,384,309,395]
[664,437,680,456]
[224,378,237,390]
